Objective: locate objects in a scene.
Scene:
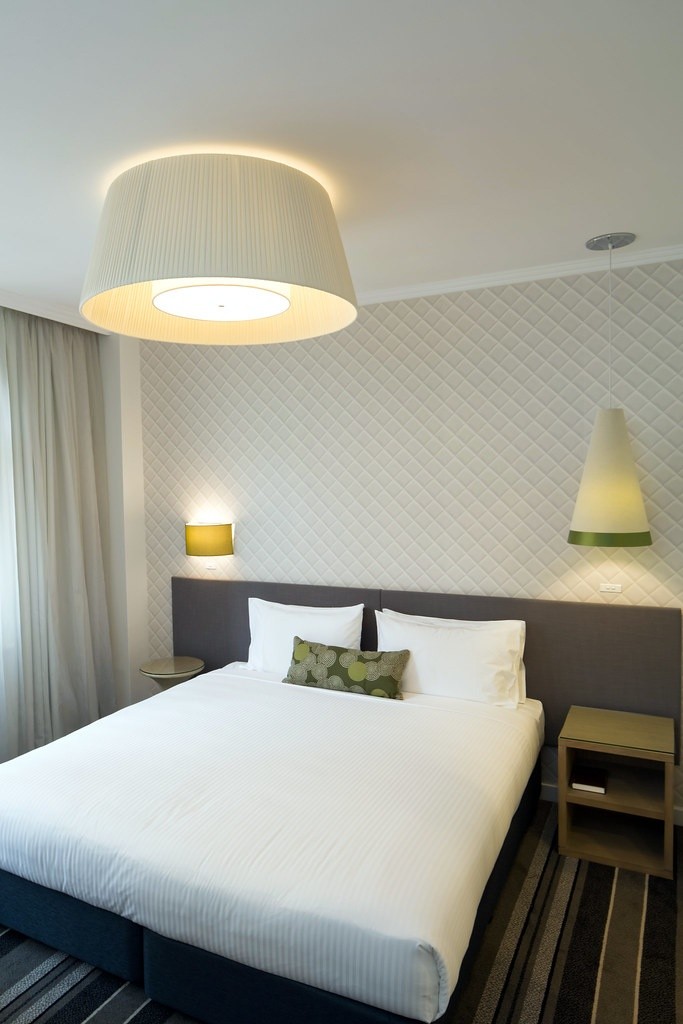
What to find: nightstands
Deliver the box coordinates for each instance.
[140,657,203,692]
[558,704,676,879]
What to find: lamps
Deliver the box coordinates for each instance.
[184,486,238,556]
[79,155,358,346]
[567,231,654,547]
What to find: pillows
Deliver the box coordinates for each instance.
[373,609,521,709]
[281,636,410,701]
[382,608,527,704]
[246,597,365,682]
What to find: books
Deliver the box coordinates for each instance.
[570,763,606,794]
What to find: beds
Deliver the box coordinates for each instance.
[0,661,543,1024]
